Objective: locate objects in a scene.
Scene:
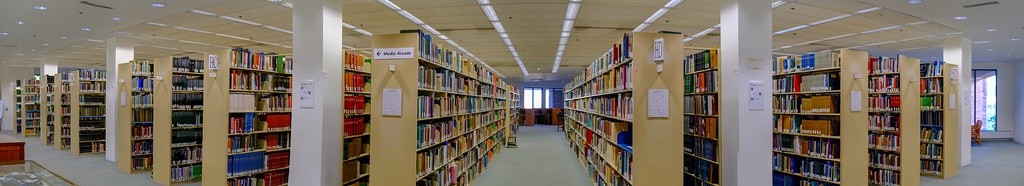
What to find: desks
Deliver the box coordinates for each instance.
[547,114,552,125]
[521,115,526,124]
[0,135,25,165]
[534,114,541,124]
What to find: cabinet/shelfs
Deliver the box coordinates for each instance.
[868,56,921,186]
[21,79,41,138]
[54,73,71,151]
[772,48,869,186]
[153,56,203,186]
[202,48,292,186]
[70,69,106,156]
[682,48,722,186]
[919,63,959,180]
[117,60,154,175]
[563,32,684,186]
[13,80,22,135]
[39,75,54,145]
[369,33,508,186]
[340,49,372,186]
[506,85,520,145]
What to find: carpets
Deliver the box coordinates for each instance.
[0,159,79,186]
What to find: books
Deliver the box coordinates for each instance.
[76,68,107,153]
[13,78,41,136]
[61,71,75,149]
[920,60,946,177]
[131,62,155,174]
[510,85,521,136]
[46,75,55,144]
[772,48,840,186]
[172,56,204,183]
[341,52,372,186]
[682,48,720,186]
[562,32,633,186]
[399,28,507,186]
[226,47,293,186]
[868,55,901,186]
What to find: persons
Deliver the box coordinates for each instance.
[557,108,564,116]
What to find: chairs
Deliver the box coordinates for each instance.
[520,113,552,126]
[557,117,565,131]
[971,120,982,147]
